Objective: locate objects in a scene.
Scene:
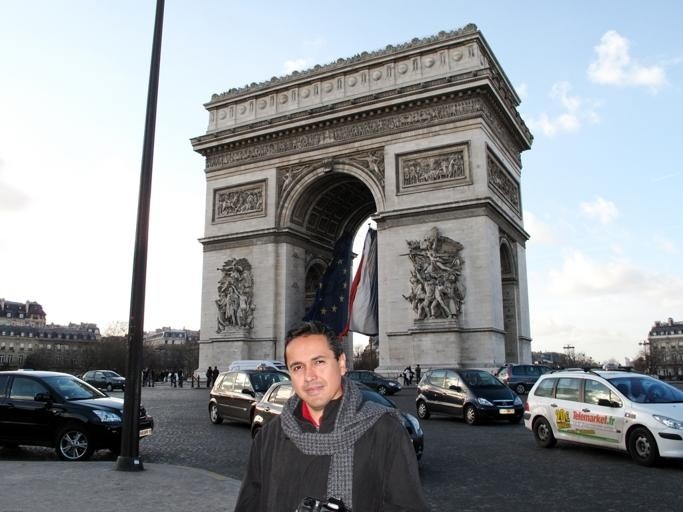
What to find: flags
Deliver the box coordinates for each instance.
[301,229,352,338]
[335,227,378,343]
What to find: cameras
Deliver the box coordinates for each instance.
[296,496,352,512]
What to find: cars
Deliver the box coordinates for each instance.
[414,367,525,425]
[522,363,683,466]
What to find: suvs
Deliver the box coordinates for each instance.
[81,369,126,392]
[0,369,155,461]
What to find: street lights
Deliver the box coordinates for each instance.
[637,339,654,373]
[563,344,576,368]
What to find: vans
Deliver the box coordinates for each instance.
[205,357,425,464]
[495,363,556,394]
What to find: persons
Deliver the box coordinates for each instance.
[278,164,310,198]
[399,154,464,187]
[140,366,218,390]
[352,150,385,180]
[232,320,429,512]
[626,383,652,403]
[397,226,469,320]
[211,259,256,335]
[396,362,421,387]
[214,187,265,215]
